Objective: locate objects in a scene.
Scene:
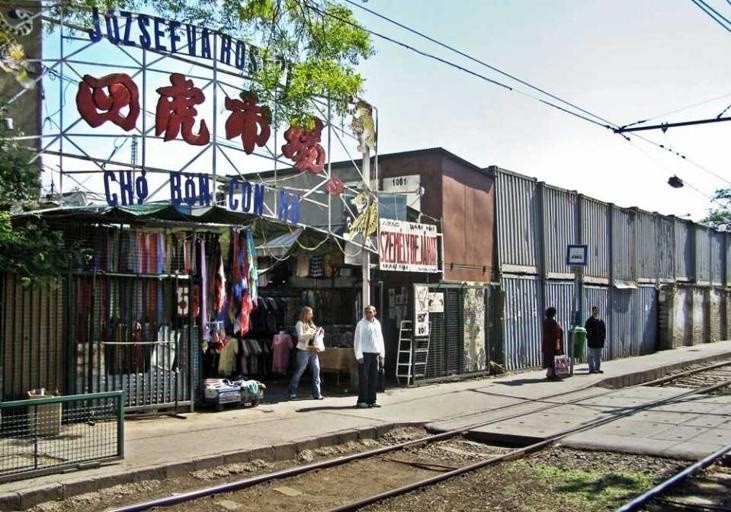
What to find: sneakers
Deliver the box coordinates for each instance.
[547,376,563,381]
[357,401,381,408]
[288,392,323,399]
[589,369,603,374]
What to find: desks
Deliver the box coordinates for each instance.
[312,346,359,388]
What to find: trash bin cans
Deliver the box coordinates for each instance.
[567,325,587,357]
[27,388,62,436]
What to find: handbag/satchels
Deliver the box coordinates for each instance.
[554,350,570,374]
[313,327,326,353]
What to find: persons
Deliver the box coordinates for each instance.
[586,306,606,374]
[353,305,384,408]
[542,306,565,379]
[288,306,325,399]
